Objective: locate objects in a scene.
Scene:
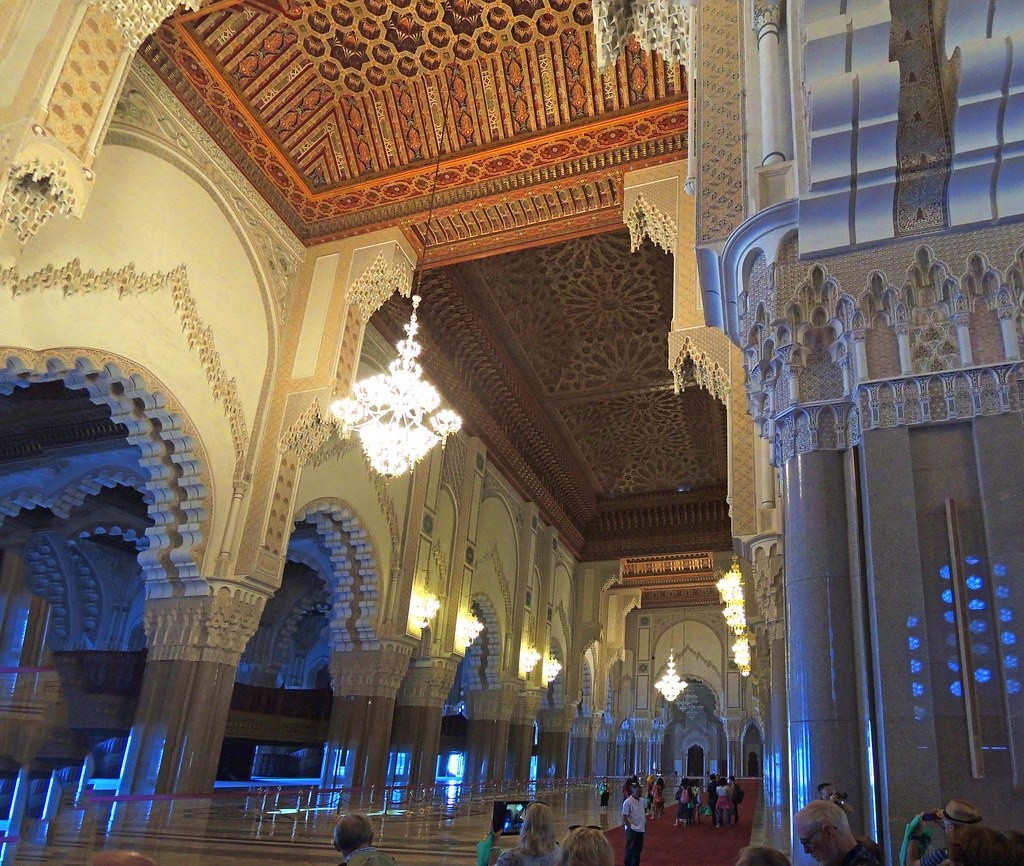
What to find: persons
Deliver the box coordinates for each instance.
[476,774,739,866]
[333,813,397,866]
[737,781,1024,866]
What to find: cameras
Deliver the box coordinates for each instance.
[833,791,849,799]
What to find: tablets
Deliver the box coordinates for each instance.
[502,801,530,835]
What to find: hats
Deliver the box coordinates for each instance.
[347,850,399,866]
[935,799,984,823]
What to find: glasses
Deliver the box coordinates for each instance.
[566,824,603,832]
[799,825,838,848]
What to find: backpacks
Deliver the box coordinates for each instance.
[733,782,744,803]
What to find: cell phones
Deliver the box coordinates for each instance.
[922,813,942,821]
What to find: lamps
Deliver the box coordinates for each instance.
[455,607,485,649]
[544,656,562,684]
[653,575,688,701]
[716,552,754,677]
[407,581,442,631]
[329,0,462,480]
[516,642,541,677]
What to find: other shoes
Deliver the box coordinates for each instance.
[682,820,703,826]
[672,824,679,827]
[714,822,738,829]
[649,815,662,820]
